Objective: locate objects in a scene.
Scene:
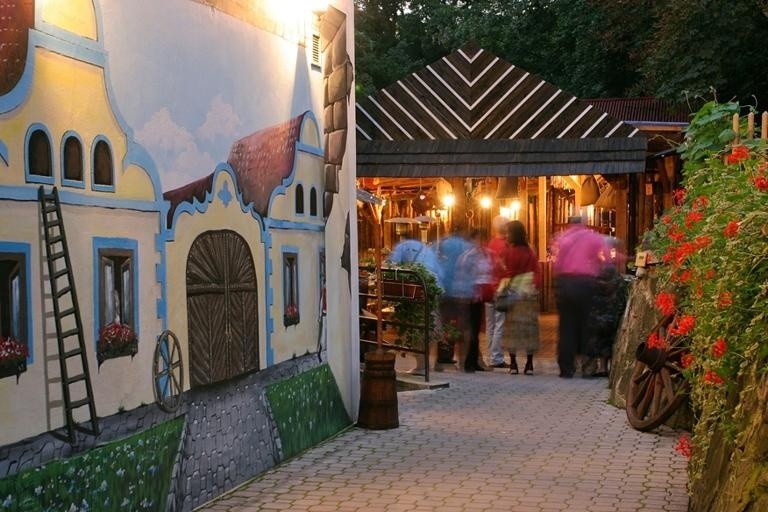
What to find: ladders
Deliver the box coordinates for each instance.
[38,185,99,443]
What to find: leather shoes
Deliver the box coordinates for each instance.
[437,354,533,375]
[560,371,608,378]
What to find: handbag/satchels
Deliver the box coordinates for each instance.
[495,288,512,311]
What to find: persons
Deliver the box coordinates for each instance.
[386,229,438,374]
[427,214,511,373]
[547,213,617,379]
[590,263,628,380]
[493,219,541,376]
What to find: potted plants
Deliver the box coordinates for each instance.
[377,262,459,358]
